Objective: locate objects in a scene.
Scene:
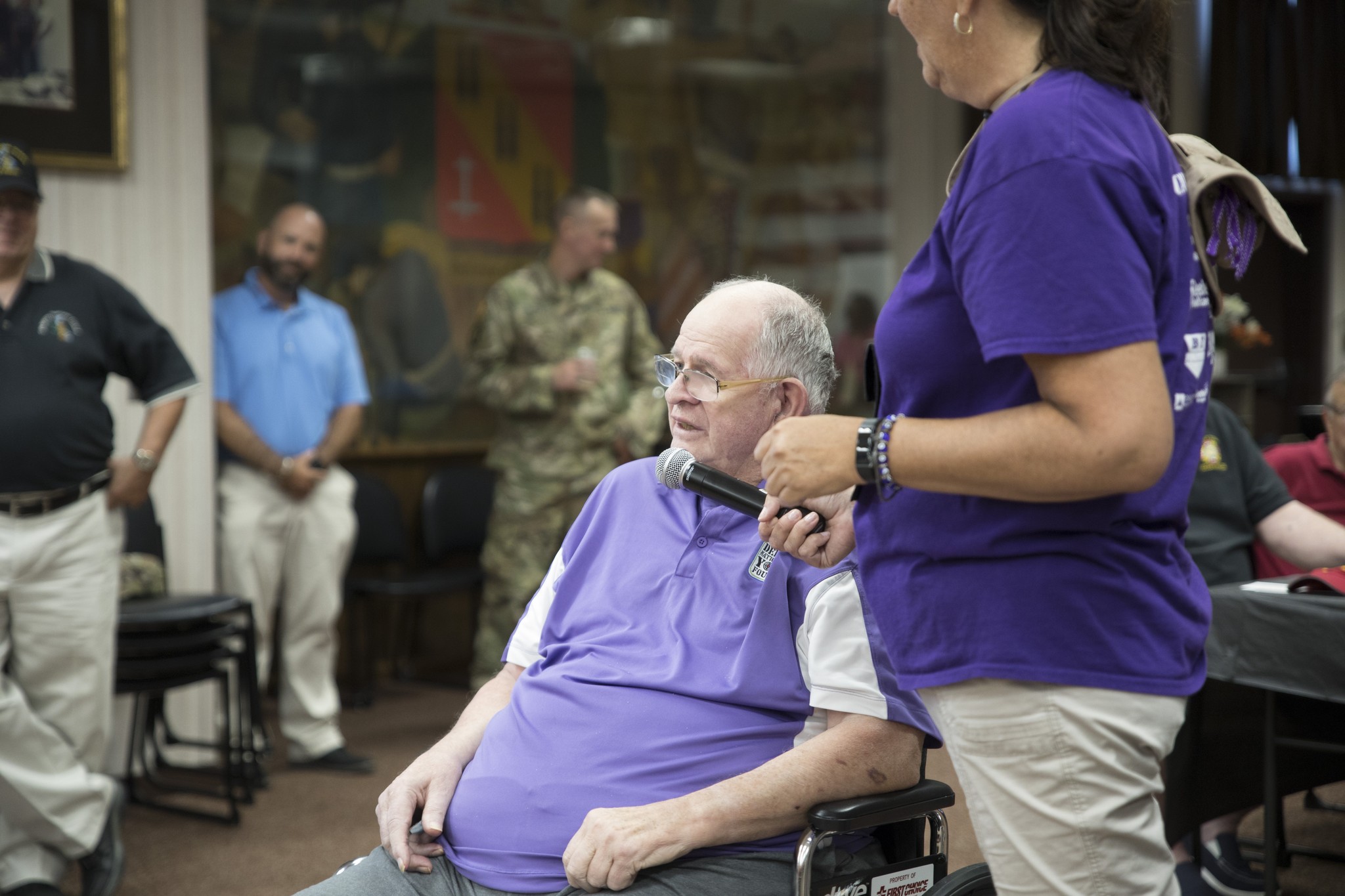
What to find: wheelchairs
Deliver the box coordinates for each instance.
[794,732,997,896]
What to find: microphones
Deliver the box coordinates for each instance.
[654,447,826,539]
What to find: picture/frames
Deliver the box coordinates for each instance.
[1,0,132,177]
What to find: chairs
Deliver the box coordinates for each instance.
[110,494,272,824]
[339,469,493,705]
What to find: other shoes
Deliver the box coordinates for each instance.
[287,747,374,772]
[77,774,126,896]
[1173,852,1220,896]
[1200,831,1283,896]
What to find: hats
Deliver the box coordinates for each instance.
[0,142,42,201]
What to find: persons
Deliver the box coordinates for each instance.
[251,0,468,438]
[462,185,665,701]
[0,154,188,896]
[1250,365,1345,578]
[753,0,1214,896]
[208,200,374,773]
[286,278,925,896]
[1167,395,1344,896]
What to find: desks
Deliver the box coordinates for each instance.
[336,404,483,663]
[1200,575,1345,875]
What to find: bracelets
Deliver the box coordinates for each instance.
[309,458,326,471]
[876,414,904,494]
[854,416,884,486]
[131,448,159,474]
[276,456,293,486]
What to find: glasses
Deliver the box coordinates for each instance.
[653,350,800,406]
